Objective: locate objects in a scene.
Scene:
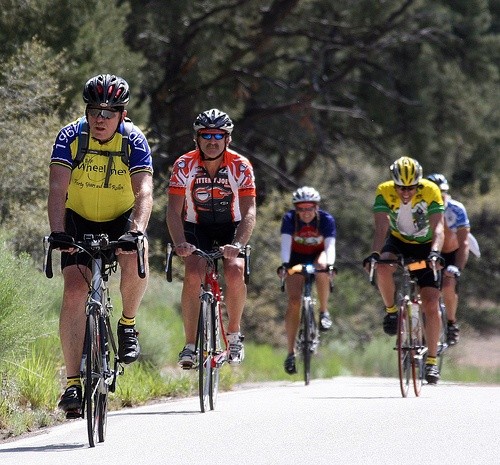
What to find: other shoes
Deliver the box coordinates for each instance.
[446,320,460,345]
[283,355,295,374]
[383,312,399,335]
[425,355,440,382]
[319,312,332,330]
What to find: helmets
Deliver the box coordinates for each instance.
[192,108,234,135]
[292,186,321,205]
[389,156,423,186]
[82,73,130,106]
[428,173,450,191]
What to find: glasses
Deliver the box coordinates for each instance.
[296,205,315,211]
[397,185,415,190]
[199,133,225,140]
[86,107,116,120]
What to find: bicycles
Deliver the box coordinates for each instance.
[370,254,457,396]
[165,242,251,413]
[42,228,145,448]
[279,263,336,387]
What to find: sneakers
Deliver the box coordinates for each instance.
[57,384,82,409]
[117,317,142,362]
[177,346,197,366]
[226,333,245,367]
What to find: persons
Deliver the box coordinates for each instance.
[277,187,336,375]
[364,157,470,380]
[47,74,155,412]
[165,108,255,369]
